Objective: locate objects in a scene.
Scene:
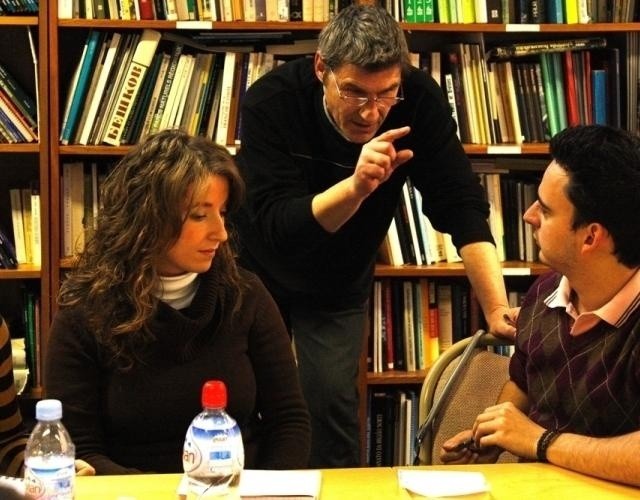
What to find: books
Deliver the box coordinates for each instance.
[367,279,529,466]
[378,160,549,266]
[0,0,40,388]
[59,163,111,286]
[58,1,640,22]
[58,28,320,146]
[408,33,638,144]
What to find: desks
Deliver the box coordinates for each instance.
[72,463,640,500]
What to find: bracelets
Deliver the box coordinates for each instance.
[537,428,567,466]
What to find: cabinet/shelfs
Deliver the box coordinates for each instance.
[0,0,640,466]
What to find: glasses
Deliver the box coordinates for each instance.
[329,66,405,107]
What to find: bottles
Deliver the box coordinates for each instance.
[23,400,75,500]
[181,379,245,500]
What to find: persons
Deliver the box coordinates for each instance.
[440,125,639,487]
[41,128,310,475]
[0,316,95,476]
[231,5,521,467]
